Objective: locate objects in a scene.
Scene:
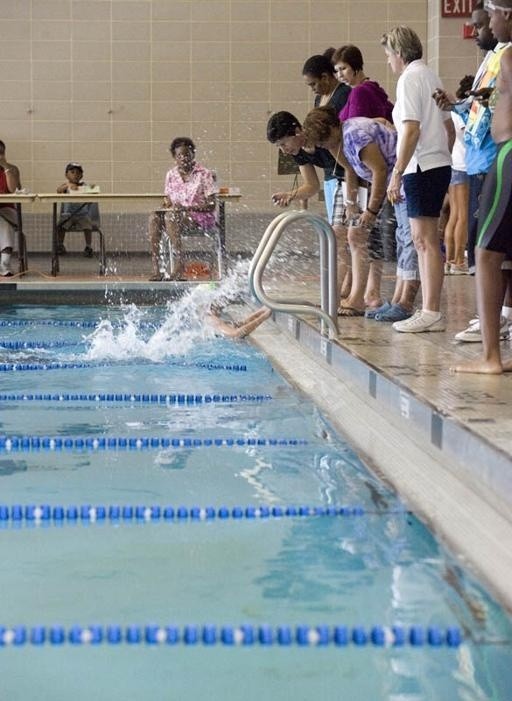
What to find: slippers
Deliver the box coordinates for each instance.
[336,305,365,318]
[374,304,413,322]
[365,303,392,320]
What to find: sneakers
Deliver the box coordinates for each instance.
[0,264,15,278]
[56,245,66,255]
[443,261,455,275]
[468,311,512,342]
[85,247,94,258]
[449,264,469,275]
[392,310,447,333]
[454,316,510,342]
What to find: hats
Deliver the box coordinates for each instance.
[65,162,85,174]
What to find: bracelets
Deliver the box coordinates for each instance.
[5,167,13,173]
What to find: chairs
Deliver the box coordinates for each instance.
[49,189,107,277]
[157,181,224,281]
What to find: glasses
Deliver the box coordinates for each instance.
[482,0,512,14]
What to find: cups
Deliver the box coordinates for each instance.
[220,187,228,194]
[228,186,241,195]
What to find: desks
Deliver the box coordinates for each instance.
[38,191,241,277]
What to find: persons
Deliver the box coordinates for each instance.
[267,0,512,373]
[56,162,94,258]
[193,285,272,342]
[147,136,217,281]
[0,140,20,275]
